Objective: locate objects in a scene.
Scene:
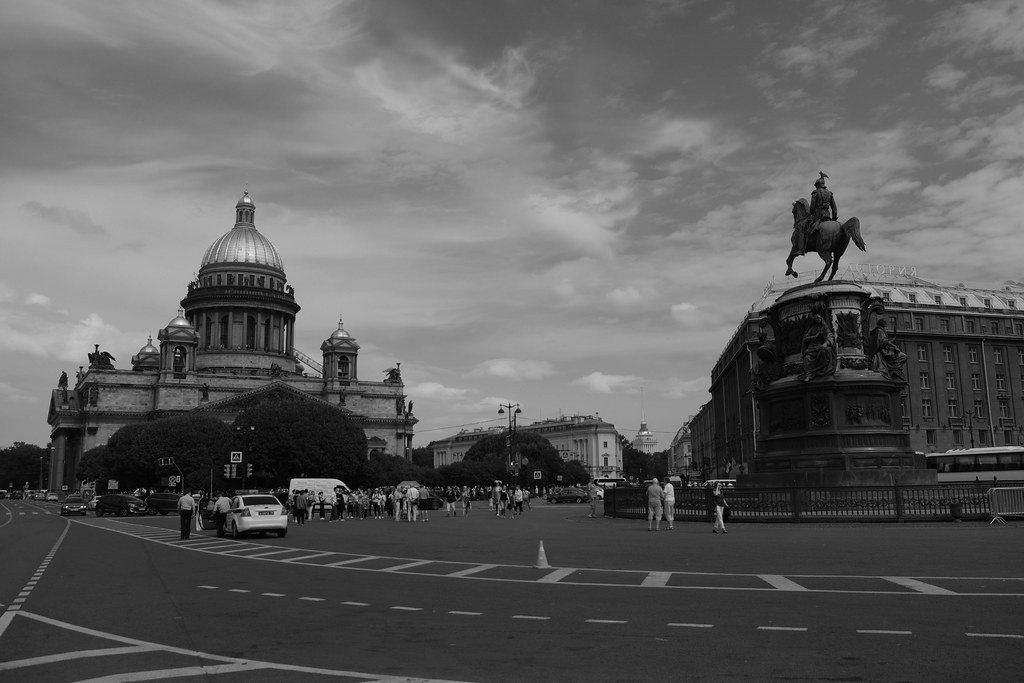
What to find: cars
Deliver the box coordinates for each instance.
[9,492,23,500]
[596,487,604,499]
[45,494,58,502]
[194,495,288,538]
[427,494,444,510]
[546,488,589,504]
[25,491,45,501]
[95,494,150,518]
[0,490,8,499]
[86,496,102,511]
[59,497,87,516]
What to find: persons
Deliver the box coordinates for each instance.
[210,493,233,537]
[712,481,728,533]
[270,480,531,525]
[806,171,839,236]
[750,332,778,387]
[802,314,835,382]
[871,318,908,380]
[133,487,157,497]
[685,479,702,488]
[645,478,676,531]
[588,483,597,518]
[548,484,575,503]
[177,487,196,539]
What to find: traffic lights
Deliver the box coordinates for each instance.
[224,465,230,478]
[248,463,252,478]
[503,463,508,473]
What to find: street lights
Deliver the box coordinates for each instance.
[498,404,522,490]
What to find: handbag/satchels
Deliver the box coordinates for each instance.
[724,507,731,517]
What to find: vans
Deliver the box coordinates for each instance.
[670,476,683,492]
[701,480,736,492]
[289,478,357,511]
[644,480,653,487]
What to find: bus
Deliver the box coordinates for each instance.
[594,478,626,488]
[915,446,1024,488]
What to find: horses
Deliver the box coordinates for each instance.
[785,198,867,282]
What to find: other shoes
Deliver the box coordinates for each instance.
[328,519,345,522]
[181,536,189,540]
[648,527,661,532]
[447,511,456,517]
[588,513,597,519]
[500,516,506,519]
[665,526,673,529]
[508,516,514,519]
[723,529,728,534]
[374,516,385,520]
[712,529,719,534]
[319,517,326,520]
[346,516,354,519]
[421,518,430,522]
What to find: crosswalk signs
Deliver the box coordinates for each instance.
[231,451,242,462]
[534,471,541,479]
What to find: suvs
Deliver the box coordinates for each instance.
[145,492,198,516]
